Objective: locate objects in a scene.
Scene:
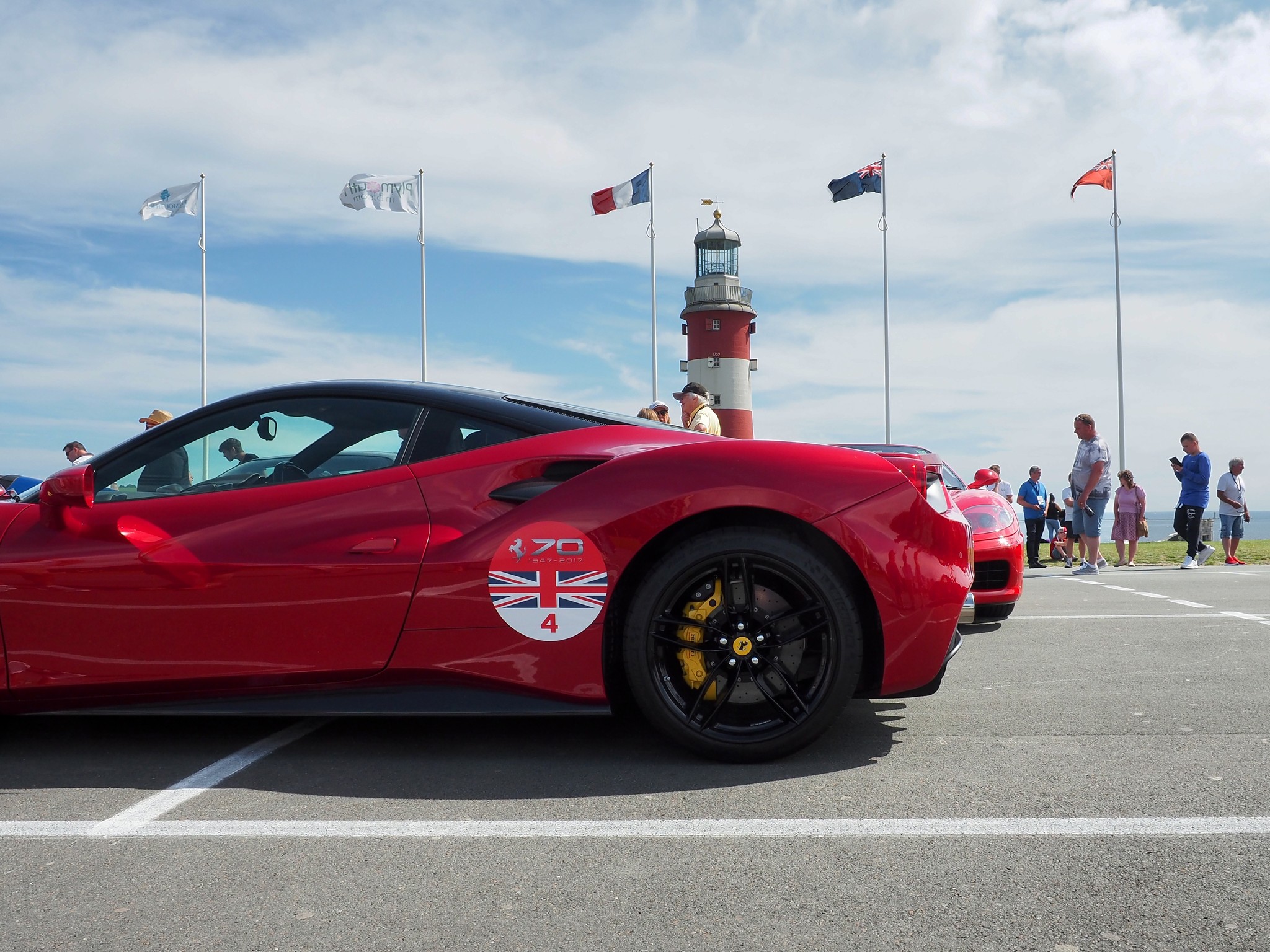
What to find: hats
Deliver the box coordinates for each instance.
[672,382,709,401]
[1057,527,1067,534]
[649,400,669,411]
[138,409,173,425]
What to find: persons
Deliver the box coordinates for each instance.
[138,409,193,493]
[636,401,671,424]
[1062,472,1088,568]
[219,437,267,477]
[1071,414,1112,575]
[62,441,119,491]
[1170,432,1215,569]
[1045,493,1062,542]
[985,465,1013,503]
[1217,458,1250,566]
[1017,466,1049,569]
[1111,470,1146,567]
[673,382,721,436]
[1050,527,1078,563]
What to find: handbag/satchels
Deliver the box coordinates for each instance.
[1136,518,1149,538]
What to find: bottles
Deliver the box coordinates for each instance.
[1081,502,1095,517]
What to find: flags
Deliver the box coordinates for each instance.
[590,168,651,216]
[138,181,202,220]
[826,157,882,203]
[339,173,420,215]
[1069,155,1113,203]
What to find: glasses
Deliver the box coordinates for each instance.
[145,423,157,427]
[681,394,689,399]
[1236,505,1247,513]
[65,447,80,456]
[1076,416,1087,422]
[657,409,668,416]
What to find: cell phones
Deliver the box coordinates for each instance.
[1040,508,1046,509]
[1246,517,1249,522]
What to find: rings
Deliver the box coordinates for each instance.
[1081,503,1083,504]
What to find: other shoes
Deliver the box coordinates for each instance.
[1080,561,1088,566]
[1064,554,1077,562]
[1027,560,1047,569]
[1064,561,1072,568]
[1113,560,1127,567]
[1128,562,1135,567]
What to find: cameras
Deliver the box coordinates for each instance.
[1082,503,1095,517]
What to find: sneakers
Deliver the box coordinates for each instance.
[1072,563,1099,575]
[1233,556,1247,566]
[1224,557,1239,566]
[1096,558,1108,569]
[1180,556,1198,569]
[1196,544,1216,566]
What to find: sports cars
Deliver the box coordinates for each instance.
[0,375,975,769]
[827,443,1024,619]
